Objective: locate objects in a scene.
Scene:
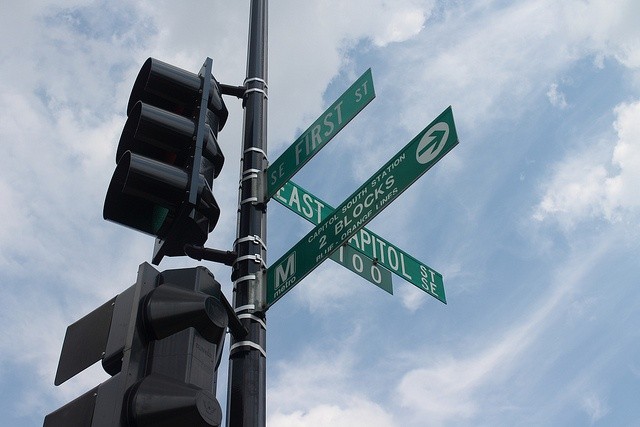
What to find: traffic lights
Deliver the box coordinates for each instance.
[103,58,238,265]
[44,262,240,427]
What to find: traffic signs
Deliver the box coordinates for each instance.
[267,68,376,202]
[264,105,458,312]
[271,180,446,304]
